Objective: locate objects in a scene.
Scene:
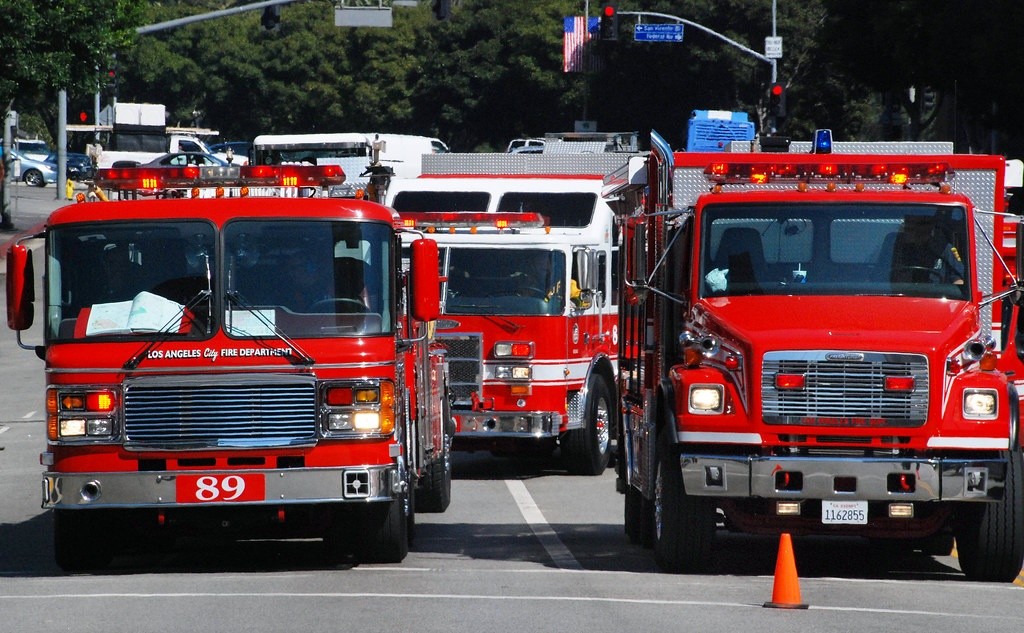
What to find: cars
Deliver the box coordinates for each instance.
[0,146,59,187]
[135,152,244,196]
[51,151,92,181]
[166,126,251,167]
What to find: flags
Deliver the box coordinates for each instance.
[563,17,607,74]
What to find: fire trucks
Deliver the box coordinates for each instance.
[618,128,1024,583]
[4,155,454,569]
[392,132,653,475]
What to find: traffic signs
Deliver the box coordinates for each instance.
[634,23,685,42]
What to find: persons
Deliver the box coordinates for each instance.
[0,144,18,231]
[872,210,964,285]
[179,144,185,153]
[514,252,592,310]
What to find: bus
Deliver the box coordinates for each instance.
[91,103,170,170]
[0,137,49,163]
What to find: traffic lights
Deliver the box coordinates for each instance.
[106,66,117,96]
[80,93,95,124]
[601,3,618,40]
[769,83,786,117]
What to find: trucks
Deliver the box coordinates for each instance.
[255,132,453,200]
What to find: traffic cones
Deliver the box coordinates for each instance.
[761,532,810,609]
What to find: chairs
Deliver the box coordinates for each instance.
[323,258,379,312]
[873,232,902,284]
[717,227,769,282]
